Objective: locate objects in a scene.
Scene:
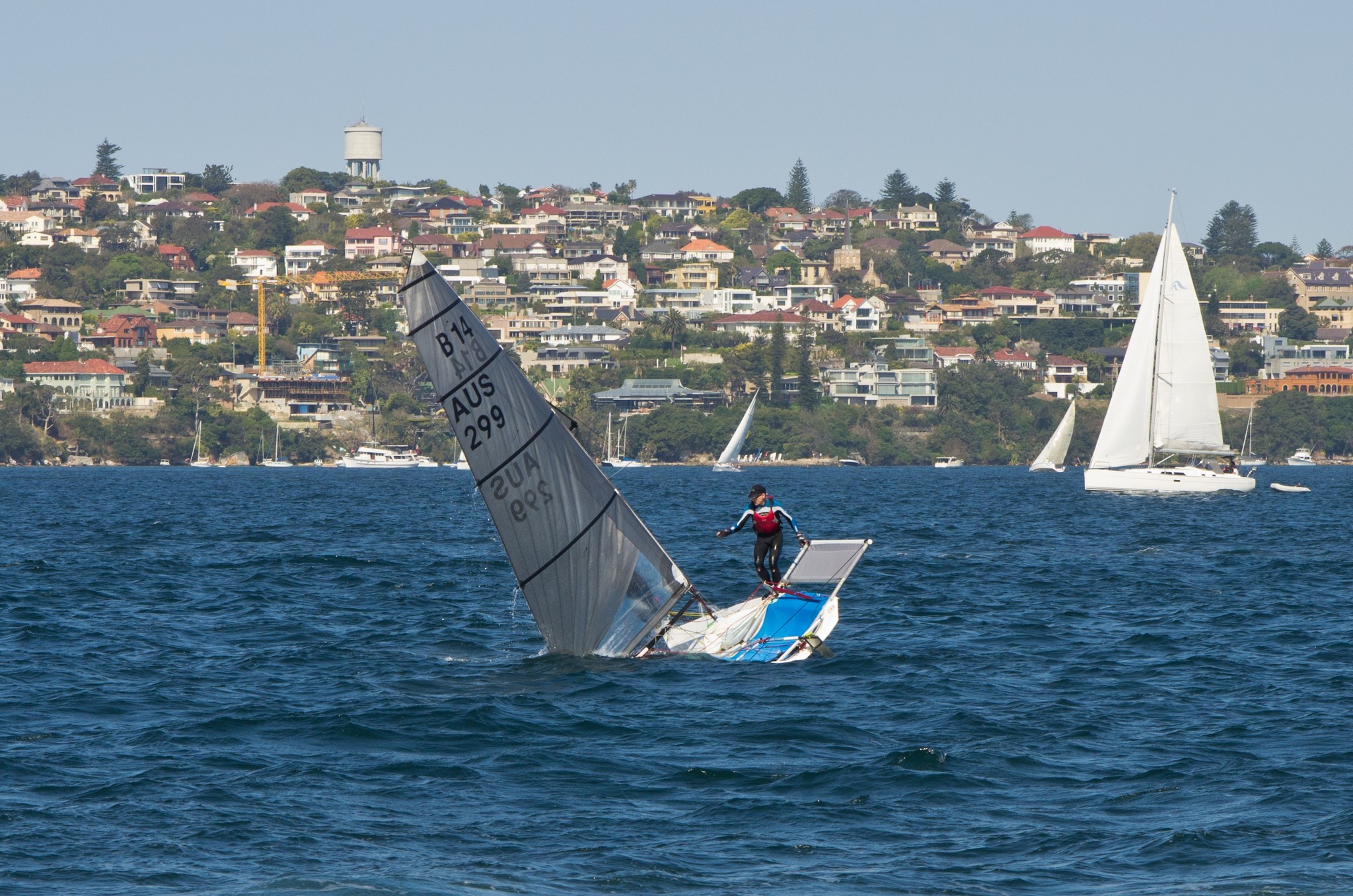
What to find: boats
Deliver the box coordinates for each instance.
[160,459,170,466]
[333,367,438,468]
[1286,443,1316,466]
[1270,483,1311,492]
[838,457,860,467]
[934,456,964,469]
[313,456,323,467]
[217,465,226,467]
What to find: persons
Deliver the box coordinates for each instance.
[1232,465,1238,475]
[1229,458,1235,467]
[717,484,807,599]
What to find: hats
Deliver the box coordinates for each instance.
[748,484,765,499]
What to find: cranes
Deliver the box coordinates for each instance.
[218,270,406,377]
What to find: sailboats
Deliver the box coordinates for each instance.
[188,420,212,467]
[256,421,293,467]
[1234,401,1267,466]
[394,246,873,663]
[711,388,761,472]
[600,409,650,467]
[1083,189,1256,497]
[1029,400,1075,472]
[439,436,471,469]
